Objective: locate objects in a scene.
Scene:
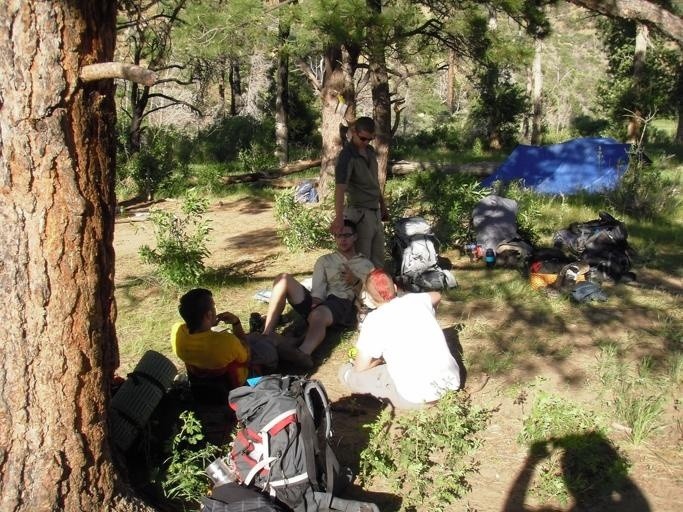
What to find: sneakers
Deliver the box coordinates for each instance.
[283,347,313,369]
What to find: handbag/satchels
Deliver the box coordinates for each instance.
[553,211,638,282]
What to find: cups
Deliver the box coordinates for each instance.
[204,456,237,486]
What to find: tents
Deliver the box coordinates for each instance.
[473,136,653,204]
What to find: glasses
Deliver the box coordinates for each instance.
[335,231,356,238]
[356,132,375,142]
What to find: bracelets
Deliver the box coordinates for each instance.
[232,320,239,325]
[351,279,360,288]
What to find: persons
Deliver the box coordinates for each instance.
[261,217,377,358]
[169,288,306,381]
[336,267,462,411]
[328,116,390,269]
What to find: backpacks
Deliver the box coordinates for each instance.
[495,236,534,269]
[229,376,342,511]
[396,217,457,292]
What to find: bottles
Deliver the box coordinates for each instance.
[485,249,496,268]
